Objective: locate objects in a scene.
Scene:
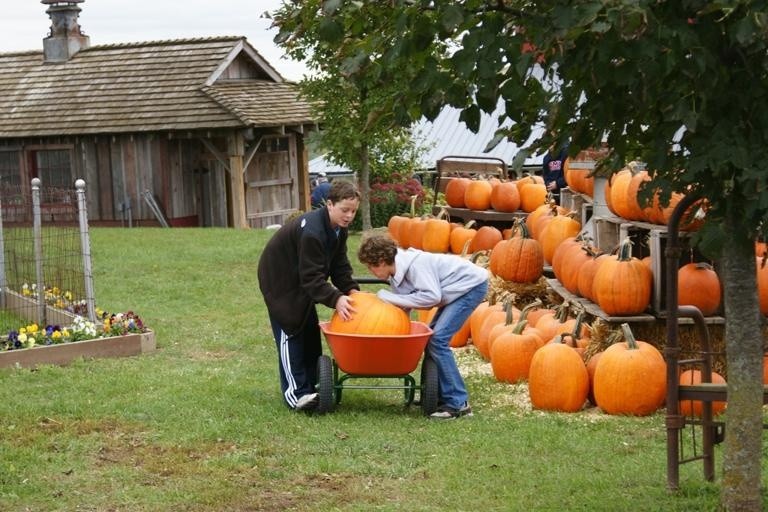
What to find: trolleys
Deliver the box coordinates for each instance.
[315,277,440,415]
[429,153,538,230]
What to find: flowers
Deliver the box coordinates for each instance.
[0,276,147,360]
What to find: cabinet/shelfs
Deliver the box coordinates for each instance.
[539,182,726,367]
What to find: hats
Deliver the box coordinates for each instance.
[317,173,327,177]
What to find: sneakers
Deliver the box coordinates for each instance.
[429,401,473,418]
[295,392,321,412]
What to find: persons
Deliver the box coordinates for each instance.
[357,229,489,420]
[312,172,334,206]
[541,132,571,206]
[309,179,318,207]
[256,178,364,413]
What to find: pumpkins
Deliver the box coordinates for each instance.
[387,149,768,416]
[328,291,412,335]
